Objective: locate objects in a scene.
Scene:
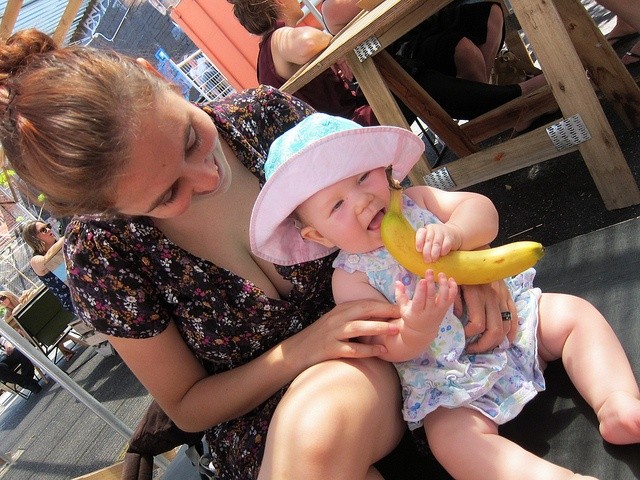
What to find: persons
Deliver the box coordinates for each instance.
[222,0,565,124]
[19,218,103,327]
[247,105,638,459]
[591,2,639,75]
[0,347,49,402]
[321,1,508,112]
[1,26,409,459]
[0,291,82,363]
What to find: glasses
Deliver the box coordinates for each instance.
[33,223,52,235]
[0,295,7,301]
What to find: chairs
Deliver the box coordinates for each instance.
[0,350,38,400]
[12,285,91,374]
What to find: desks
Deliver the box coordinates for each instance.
[273,0,640,210]
[5,290,39,335]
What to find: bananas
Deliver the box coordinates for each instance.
[378,163,547,289]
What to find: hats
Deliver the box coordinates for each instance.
[249,110,426,269]
